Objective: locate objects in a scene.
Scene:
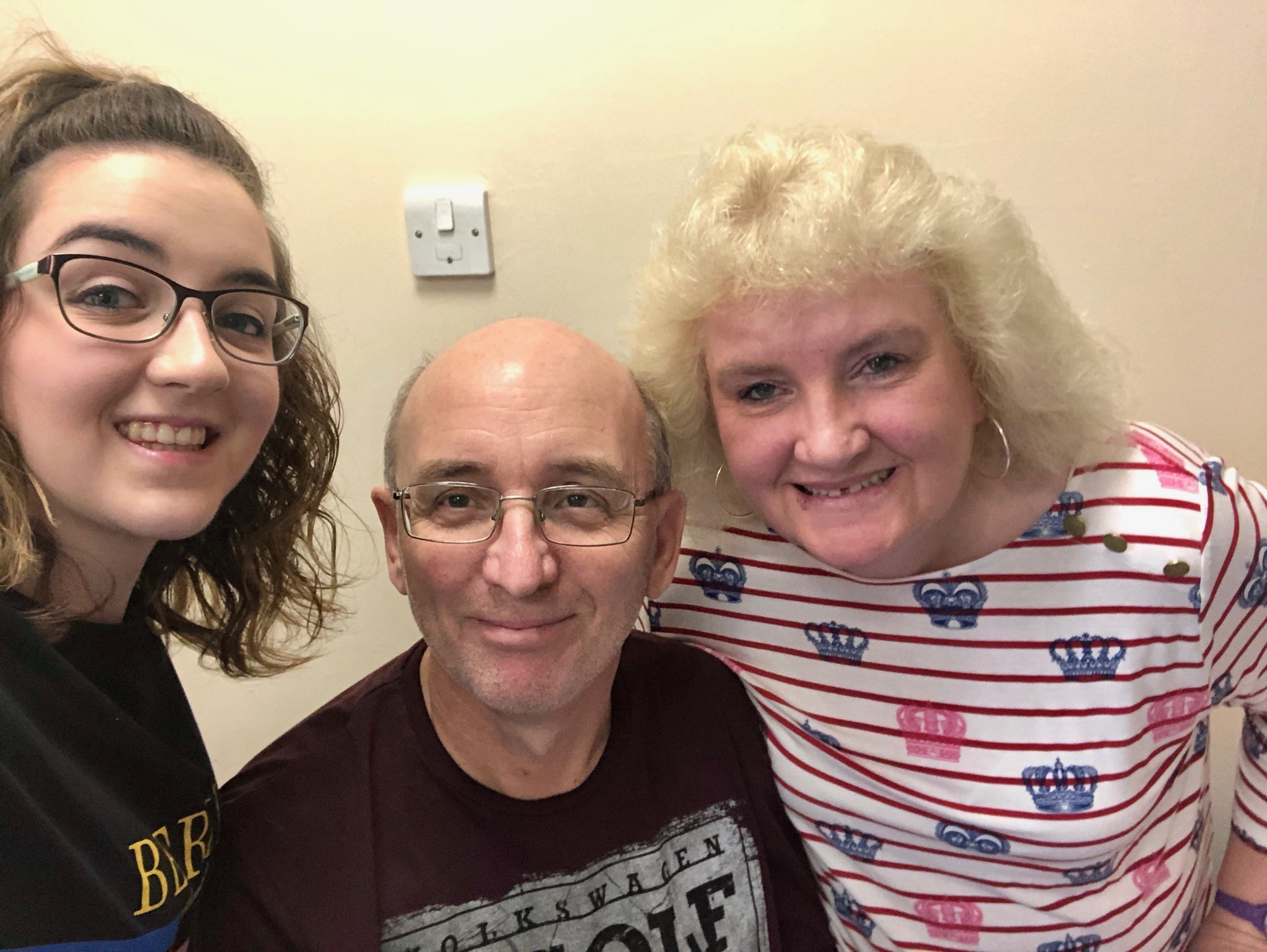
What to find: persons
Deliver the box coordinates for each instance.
[0,53,352,952]
[606,126,1267,952]
[188,319,836,952]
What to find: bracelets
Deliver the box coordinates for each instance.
[1210,888,1267,936]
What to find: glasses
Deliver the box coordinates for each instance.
[393,482,655,547]
[3,253,309,366]
